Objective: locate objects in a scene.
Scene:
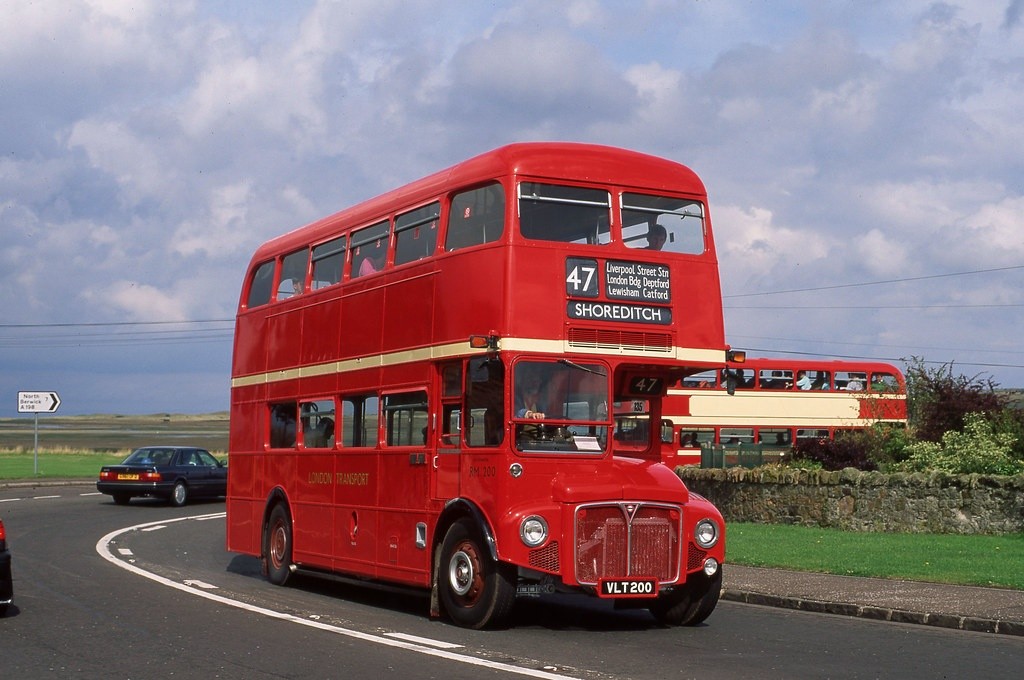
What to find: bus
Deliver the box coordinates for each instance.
[226,138,746,632]
[613,356,909,470]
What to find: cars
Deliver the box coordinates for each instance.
[96,446,228,505]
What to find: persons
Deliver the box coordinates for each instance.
[358,244,387,276]
[642,224,667,251]
[291,417,334,448]
[719,368,892,392]
[513,379,548,421]
[286,272,304,299]
[619,423,785,445]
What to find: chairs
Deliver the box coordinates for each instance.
[484,410,501,445]
